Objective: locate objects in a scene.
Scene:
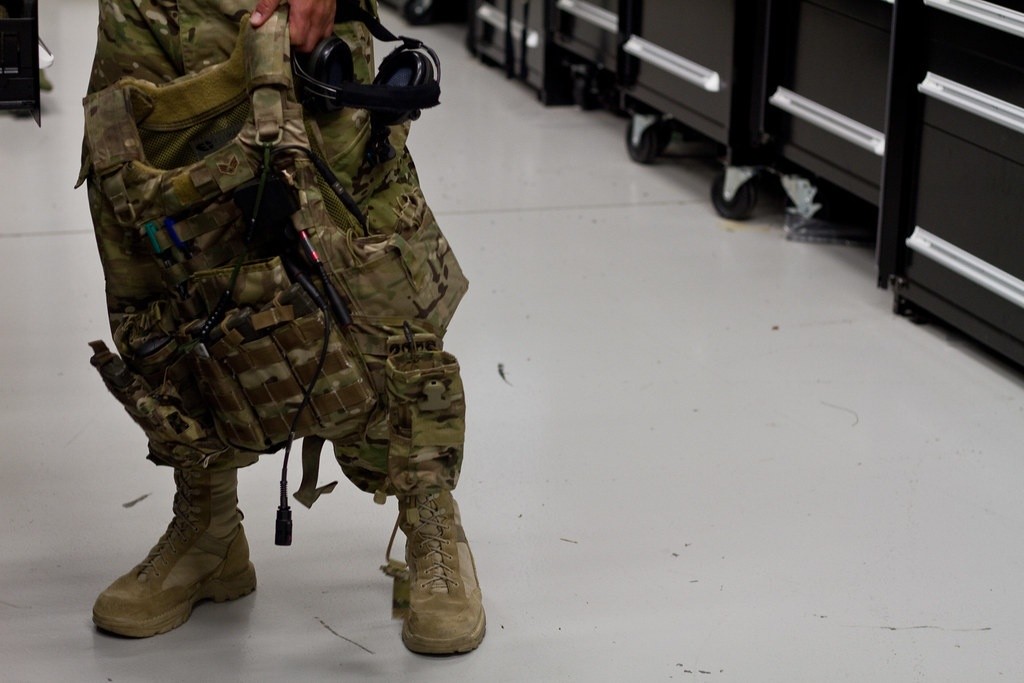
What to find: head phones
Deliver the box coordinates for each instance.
[287,31,442,129]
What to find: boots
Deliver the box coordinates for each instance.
[91,467,256,638]
[381,482,486,654]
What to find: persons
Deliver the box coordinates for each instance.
[76,0,485,655]
